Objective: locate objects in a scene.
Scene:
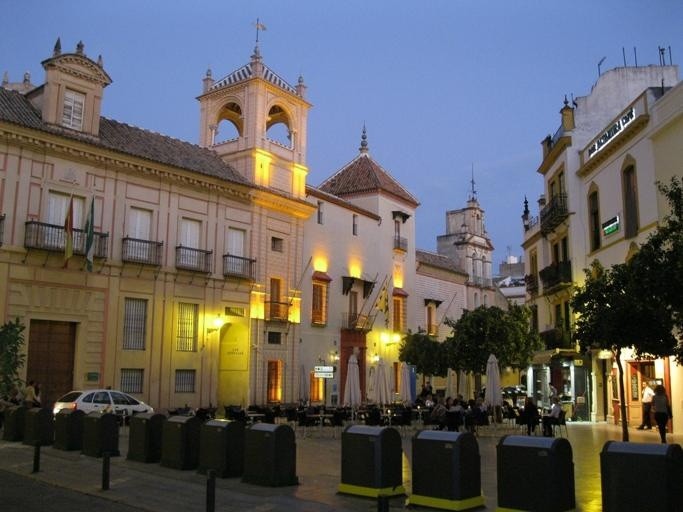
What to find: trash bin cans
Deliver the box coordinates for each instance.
[577,396,586,421]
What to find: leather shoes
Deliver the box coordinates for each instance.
[636,425,653,430]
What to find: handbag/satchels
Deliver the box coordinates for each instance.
[666,407,673,419]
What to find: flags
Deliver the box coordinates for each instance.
[86,198,94,274]
[63,196,74,269]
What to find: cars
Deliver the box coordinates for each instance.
[53,389,155,426]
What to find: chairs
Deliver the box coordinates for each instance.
[167,397,568,438]
[95,394,108,402]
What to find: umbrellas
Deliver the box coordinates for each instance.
[342,354,362,424]
[374,357,392,416]
[484,353,503,424]
[401,361,412,410]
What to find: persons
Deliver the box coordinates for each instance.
[414,382,488,433]
[636,381,656,431]
[7,380,44,409]
[650,385,673,443]
[523,383,564,437]
